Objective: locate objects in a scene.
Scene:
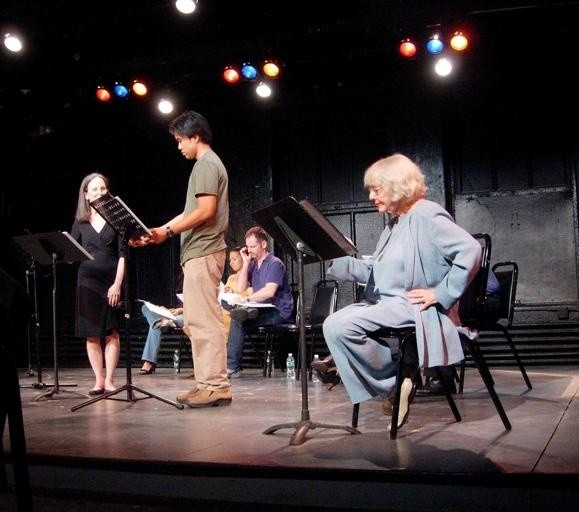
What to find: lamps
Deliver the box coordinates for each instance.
[95,79,148,104]
[221,59,281,85]
[399,22,469,58]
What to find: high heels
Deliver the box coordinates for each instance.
[139,363,156,375]
[159,318,178,334]
[311,355,340,392]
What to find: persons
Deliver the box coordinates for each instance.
[128,110,233,408]
[222,246,250,344]
[326,153,483,431]
[138,304,184,375]
[71,172,128,396]
[220,226,294,379]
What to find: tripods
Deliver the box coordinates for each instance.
[19,257,81,392]
[70,233,185,414]
[32,254,90,402]
[262,251,361,444]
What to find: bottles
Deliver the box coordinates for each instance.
[285,352,296,382]
[266,351,275,375]
[173,348,180,375]
[311,354,321,382]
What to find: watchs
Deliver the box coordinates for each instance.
[166,227,174,238]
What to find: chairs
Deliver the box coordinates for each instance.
[352,232,512,440]
[426,261,532,395]
[175,279,339,382]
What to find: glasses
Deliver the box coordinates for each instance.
[87,186,107,194]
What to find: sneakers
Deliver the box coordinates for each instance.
[226,369,242,379]
[177,385,200,404]
[230,307,259,321]
[185,388,232,409]
[387,375,414,435]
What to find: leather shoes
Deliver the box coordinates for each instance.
[415,379,457,398]
[89,383,115,394]
